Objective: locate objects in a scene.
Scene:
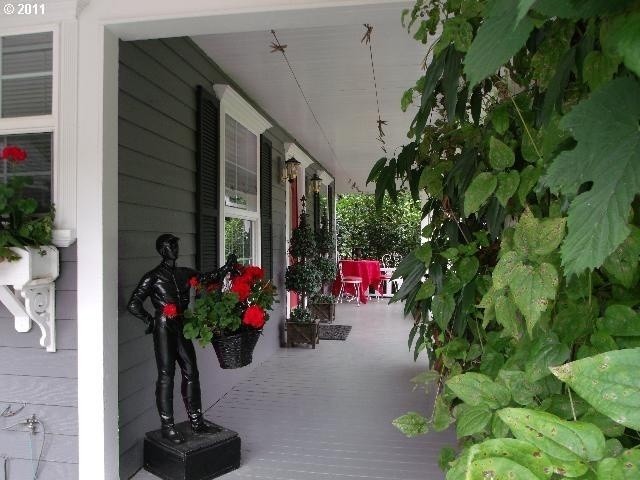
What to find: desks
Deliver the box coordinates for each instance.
[333,260,383,304]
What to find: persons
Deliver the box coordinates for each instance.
[127,233,239,445]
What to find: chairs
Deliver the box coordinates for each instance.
[374,252,402,300]
[336,262,362,306]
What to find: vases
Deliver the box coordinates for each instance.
[211,330,260,369]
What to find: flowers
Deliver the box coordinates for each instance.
[0,144,55,263]
[163,263,282,348]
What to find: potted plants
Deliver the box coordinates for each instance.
[284,195,338,350]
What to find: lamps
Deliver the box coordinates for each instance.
[306,172,322,197]
[281,155,302,183]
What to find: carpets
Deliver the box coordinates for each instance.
[318,325,353,340]
